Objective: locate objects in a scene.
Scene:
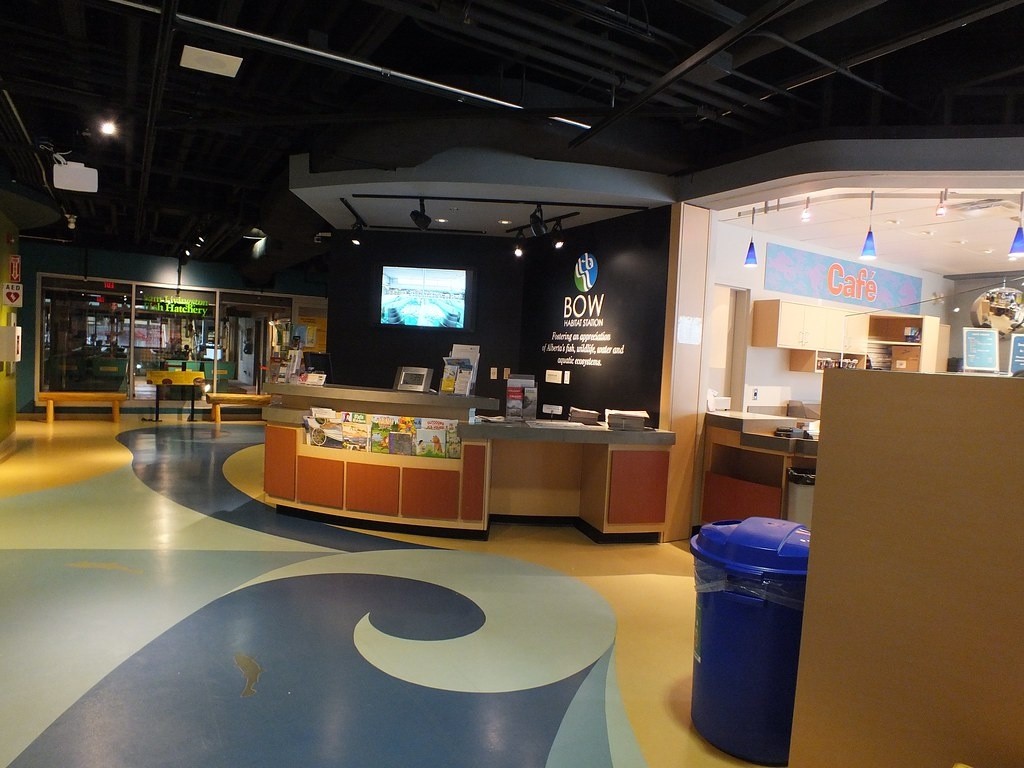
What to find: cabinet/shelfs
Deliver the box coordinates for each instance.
[789,349,867,373]
[866,313,941,374]
[751,299,844,352]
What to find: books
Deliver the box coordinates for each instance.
[798,419,820,440]
[569,407,598,424]
[302,408,461,459]
[605,409,645,430]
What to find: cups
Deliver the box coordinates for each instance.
[818,358,839,369]
[842,359,858,369]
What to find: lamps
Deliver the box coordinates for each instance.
[179,249,191,266]
[1008,191,1024,257]
[550,219,568,249]
[744,207,759,268]
[52,150,99,193]
[409,198,433,230]
[859,191,877,261]
[936,191,946,217]
[350,222,364,247]
[530,208,548,237]
[242,227,268,240]
[194,231,209,248]
[801,197,811,223]
[512,229,526,258]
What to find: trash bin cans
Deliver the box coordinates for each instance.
[788,468,817,527]
[690,517,810,768]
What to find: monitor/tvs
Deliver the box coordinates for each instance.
[380,266,466,329]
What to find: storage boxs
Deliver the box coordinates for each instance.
[891,345,921,372]
[605,409,645,431]
[568,408,599,425]
[715,396,731,409]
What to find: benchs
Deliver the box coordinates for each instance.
[206,393,272,422]
[37,391,127,425]
